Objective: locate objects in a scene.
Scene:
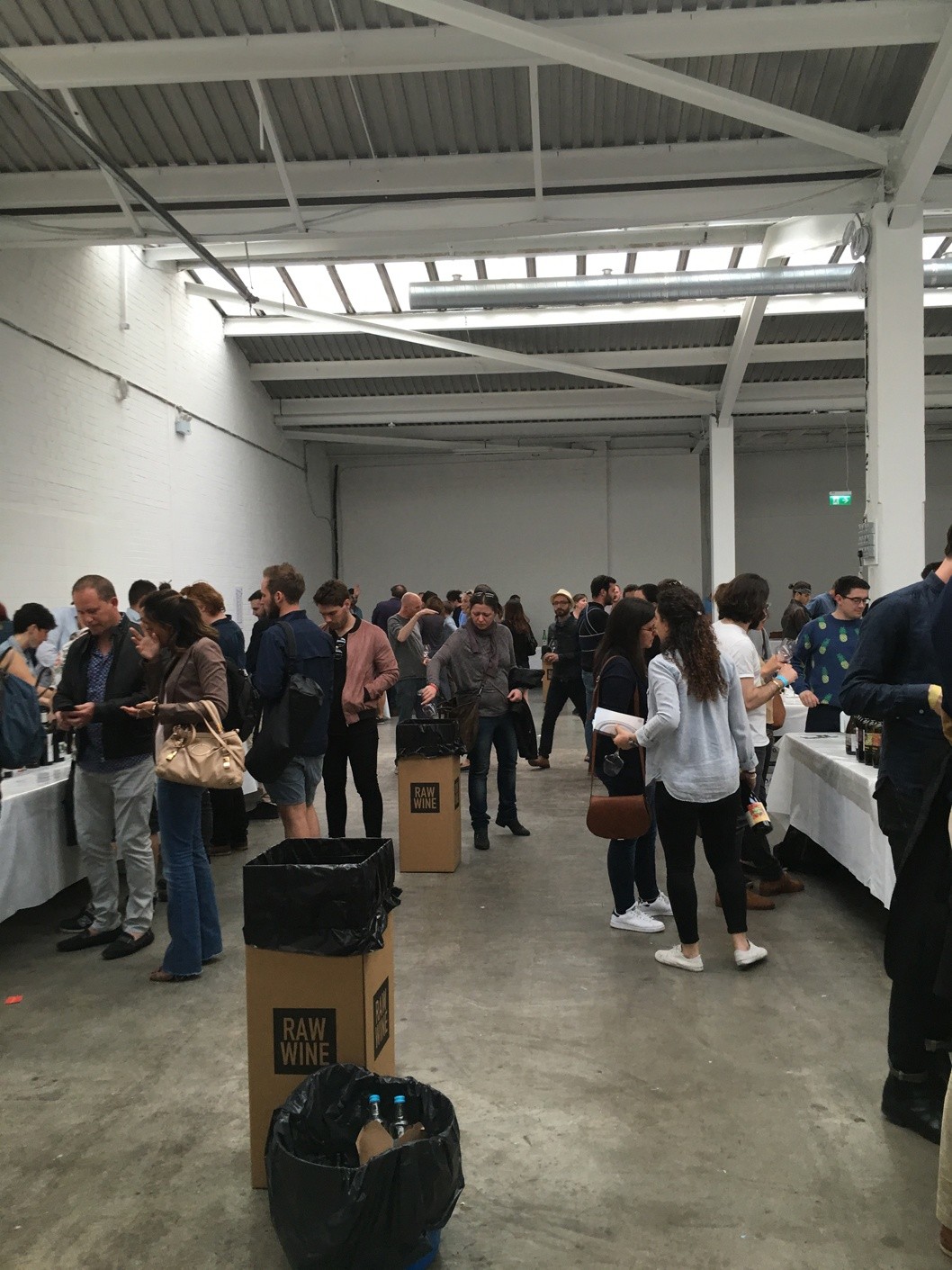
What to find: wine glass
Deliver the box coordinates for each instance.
[604,746,624,778]
[548,640,558,653]
[416,688,437,717]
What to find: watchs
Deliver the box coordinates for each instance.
[629,735,640,749]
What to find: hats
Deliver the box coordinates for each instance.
[550,588,574,609]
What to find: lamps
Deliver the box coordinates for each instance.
[175,413,192,435]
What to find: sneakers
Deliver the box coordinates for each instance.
[655,944,703,972]
[636,890,673,916]
[610,901,665,933]
[734,938,768,966]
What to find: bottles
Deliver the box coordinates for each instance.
[542,630,548,646]
[389,1095,410,1138]
[845,714,885,768]
[364,1094,390,1135]
[38,704,77,765]
[739,780,773,836]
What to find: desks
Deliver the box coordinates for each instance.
[766,733,896,910]
[0,741,124,923]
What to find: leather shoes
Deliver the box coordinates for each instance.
[881,1071,945,1145]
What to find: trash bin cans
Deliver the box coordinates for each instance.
[541,645,554,703]
[243,837,394,1188]
[396,719,462,873]
[270,1076,457,1270]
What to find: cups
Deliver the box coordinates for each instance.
[421,644,431,658]
[776,638,798,664]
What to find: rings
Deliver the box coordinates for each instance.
[137,715,139,719]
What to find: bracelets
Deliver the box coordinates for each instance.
[741,772,757,780]
[430,683,438,692]
[770,678,781,690]
[776,674,788,687]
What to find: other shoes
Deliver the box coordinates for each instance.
[759,872,804,897]
[528,755,550,768]
[715,888,775,910]
[247,803,279,819]
[940,1226,952,1258]
[496,816,530,836]
[584,751,590,762]
[473,828,489,849]
[57,839,248,982]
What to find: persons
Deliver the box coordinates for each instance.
[936,574,952,1259]
[119,583,229,982]
[249,563,336,839]
[613,579,768,973]
[0,562,942,930]
[313,578,399,839]
[839,526,952,1144]
[591,597,673,933]
[421,584,530,850]
[53,575,155,960]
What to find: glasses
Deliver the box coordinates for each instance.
[842,595,870,605]
[552,601,569,606]
[473,592,495,598]
[642,627,655,632]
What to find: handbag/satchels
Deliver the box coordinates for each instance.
[765,691,786,730]
[586,794,651,839]
[0,648,54,769]
[153,699,246,789]
[449,694,480,755]
[262,623,323,753]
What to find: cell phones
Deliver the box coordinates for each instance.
[57,703,79,719]
